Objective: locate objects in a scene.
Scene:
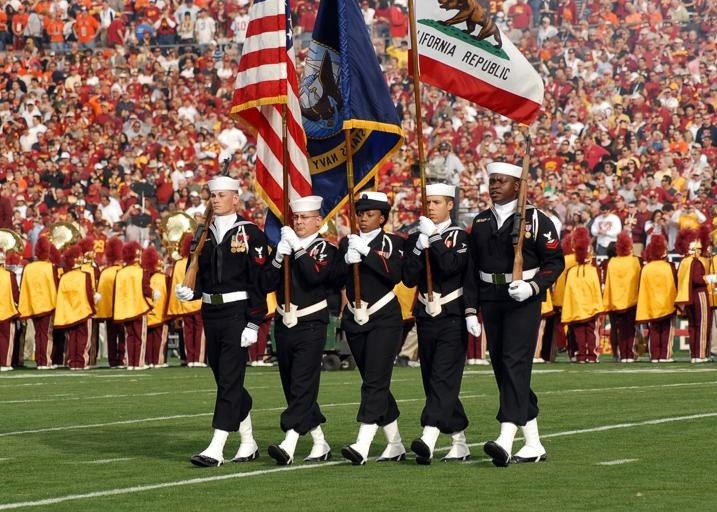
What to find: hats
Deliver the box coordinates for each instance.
[288,195,323,213]
[208,176,240,191]
[561,226,715,262]
[5,231,192,271]
[485,162,523,179]
[359,191,387,202]
[425,183,456,197]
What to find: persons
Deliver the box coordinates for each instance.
[329,191,407,466]
[399,182,471,465]
[253,195,339,466]
[186,175,270,468]
[461,161,565,467]
[1,0,717,370]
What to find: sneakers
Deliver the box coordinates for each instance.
[375,452,407,462]
[190,454,226,465]
[268,443,293,465]
[302,449,331,462]
[250,360,273,367]
[512,453,548,463]
[230,448,260,463]
[411,438,432,464]
[484,442,511,467]
[108,361,208,371]
[439,453,470,462]
[342,445,366,465]
[466,359,489,366]
[0,363,92,372]
[531,355,712,365]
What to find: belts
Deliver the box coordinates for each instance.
[276,300,328,319]
[478,267,540,285]
[200,291,248,306]
[347,292,396,319]
[418,287,463,309]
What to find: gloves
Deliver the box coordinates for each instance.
[506,280,534,302]
[175,283,194,301]
[415,215,436,251]
[274,224,304,264]
[240,327,259,347]
[344,233,369,264]
[465,316,482,337]
